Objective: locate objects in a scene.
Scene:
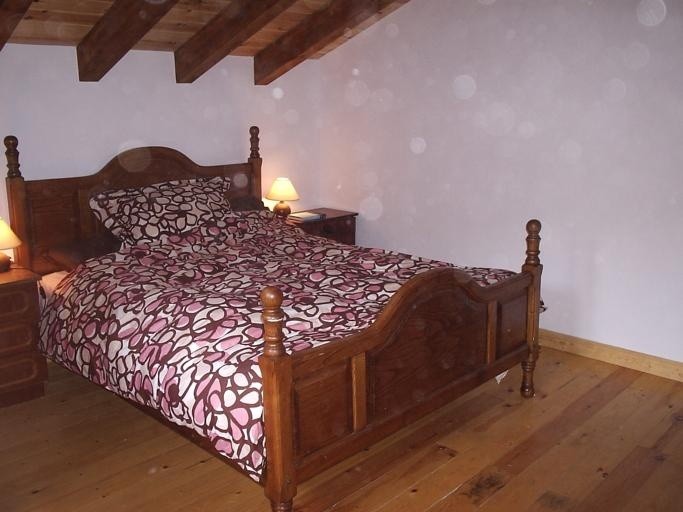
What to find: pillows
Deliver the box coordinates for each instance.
[88,174,233,252]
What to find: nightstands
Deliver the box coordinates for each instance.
[1,261,47,409]
[287,208,358,246]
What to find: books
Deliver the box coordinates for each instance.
[288,211,326,222]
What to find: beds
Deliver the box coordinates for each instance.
[3,125,544,512]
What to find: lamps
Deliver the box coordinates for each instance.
[265,177,299,219]
[0,216,23,273]
